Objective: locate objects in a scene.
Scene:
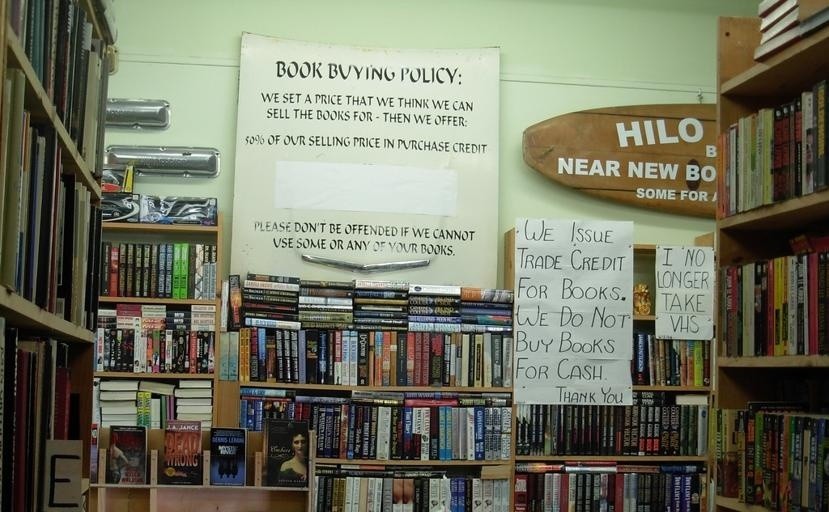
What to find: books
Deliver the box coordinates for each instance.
[1,0,105,511]
[88,244,217,484]
[210,272,708,512]
[707,1,828,512]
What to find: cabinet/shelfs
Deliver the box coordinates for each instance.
[0,2,829,510]
